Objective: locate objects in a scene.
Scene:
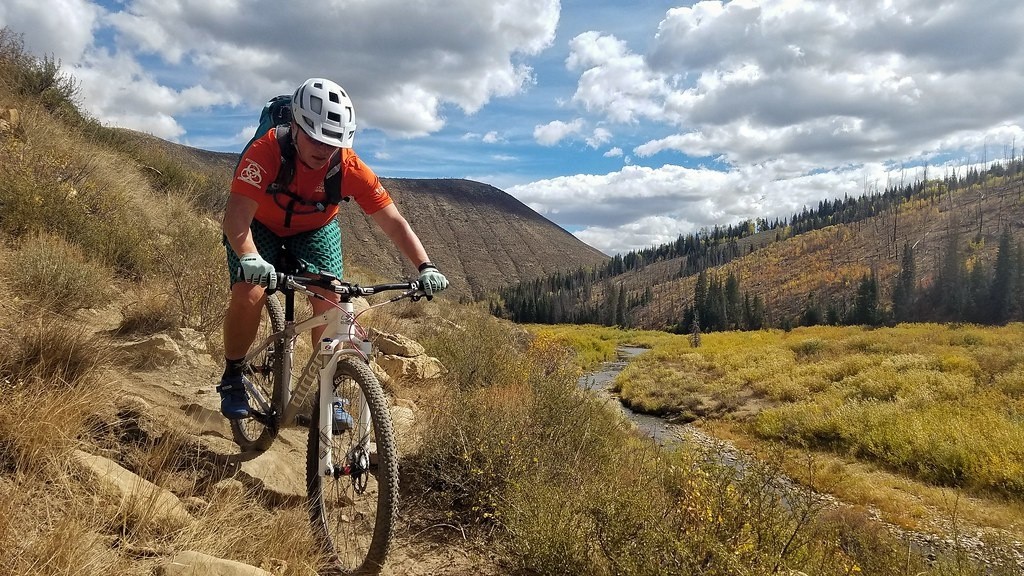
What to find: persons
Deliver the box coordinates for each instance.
[215,77,446,429]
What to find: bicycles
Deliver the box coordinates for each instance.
[229,257,451,576]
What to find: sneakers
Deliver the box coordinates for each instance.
[216,377,251,419]
[332,392,353,430]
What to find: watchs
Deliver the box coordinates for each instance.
[418,262,436,272]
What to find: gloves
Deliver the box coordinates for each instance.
[417,262,448,301]
[239,253,277,295]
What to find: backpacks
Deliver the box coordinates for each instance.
[240,95,342,205]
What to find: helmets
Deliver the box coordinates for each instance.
[291,77,356,148]
